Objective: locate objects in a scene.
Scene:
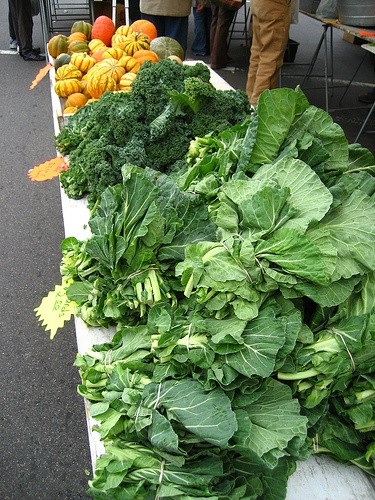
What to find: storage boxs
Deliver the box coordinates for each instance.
[343,31,363,45]
[284,38,300,62]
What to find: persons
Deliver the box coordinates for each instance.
[116,0,141,24]
[246,0,299,110]
[191,0,245,70]
[139,0,207,59]
[8,0,46,61]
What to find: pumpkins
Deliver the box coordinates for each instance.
[48,15,185,115]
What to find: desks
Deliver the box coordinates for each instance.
[242,7,375,143]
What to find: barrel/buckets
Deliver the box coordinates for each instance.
[299,0,321,14]
[338,0,375,27]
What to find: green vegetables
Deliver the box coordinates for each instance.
[59,83,375,500]
[55,58,255,218]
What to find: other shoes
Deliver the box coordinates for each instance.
[24,53,46,61]
[18,47,41,57]
[10,40,17,49]
